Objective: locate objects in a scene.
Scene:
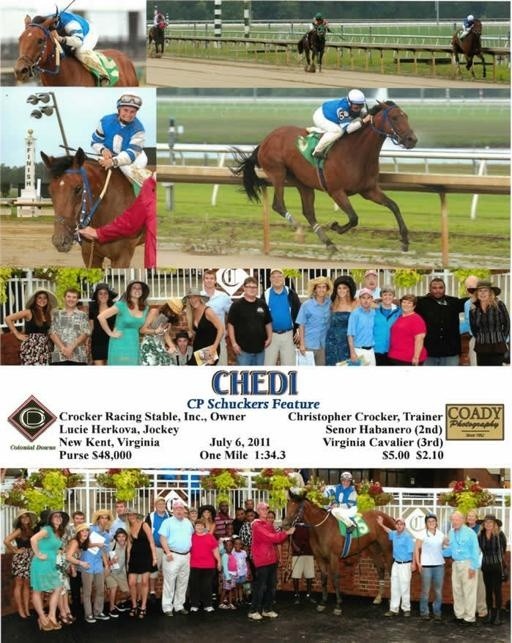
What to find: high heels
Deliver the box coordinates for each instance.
[25,611,32,618]
[58,613,72,624]
[47,616,62,629]
[18,613,27,620]
[67,612,76,620]
[37,617,53,630]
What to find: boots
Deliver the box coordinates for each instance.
[494,608,501,625]
[483,608,494,623]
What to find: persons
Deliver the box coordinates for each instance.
[347,287,377,365]
[3,507,37,619]
[295,275,333,365]
[124,507,157,617]
[249,502,296,615]
[91,94,149,186]
[200,270,233,365]
[31,10,109,87]
[466,509,482,535]
[233,506,245,534]
[88,283,118,364]
[138,298,183,365]
[383,293,427,365]
[109,499,129,537]
[48,288,91,365]
[80,171,156,268]
[183,287,224,365]
[170,329,193,365]
[289,521,316,604]
[260,267,302,365]
[417,277,469,365]
[459,14,474,38]
[467,278,511,365]
[158,500,194,615]
[357,270,382,299]
[30,510,69,629]
[366,480,382,499]
[313,13,328,29]
[145,496,172,603]
[105,528,130,618]
[187,507,198,525]
[67,510,85,537]
[97,280,149,365]
[377,515,417,616]
[191,519,222,611]
[155,11,164,25]
[200,504,218,528]
[227,277,273,365]
[266,510,282,603]
[79,508,111,622]
[219,539,238,608]
[415,511,449,620]
[219,522,239,551]
[56,523,90,623]
[325,275,357,365]
[311,88,370,158]
[245,498,255,513]
[376,284,403,365]
[213,500,234,536]
[477,513,507,625]
[441,509,481,625]
[460,276,485,365]
[5,286,58,365]
[231,537,248,603]
[241,508,256,562]
[324,471,359,533]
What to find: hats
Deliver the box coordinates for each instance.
[93,508,111,521]
[13,508,37,527]
[39,510,52,525]
[476,513,502,526]
[155,496,165,502]
[182,287,210,303]
[118,507,144,521]
[173,501,184,507]
[308,275,333,294]
[125,279,149,299]
[26,287,57,308]
[92,283,117,299]
[395,517,405,523]
[114,527,127,540]
[365,269,376,276]
[167,296,183,313]
[271,267,282,273]
[47,509,69,527]
[332,275,356,300]
[173,329,192,343]
[74,523,89,534]
[359,287,371,296]
[257,501,269,509]
[199,504,216,517]
[468,280,501,295]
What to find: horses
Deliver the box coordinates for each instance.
[38,147,148,269]
[12,12,139,85]
[452,18,485,85]
[282,485,400,616]
[149,21,166,56]
[225,97,418,258]
[296,22,327,73]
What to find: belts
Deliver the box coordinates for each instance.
[169,549,190,554]
[361,346,372,349]
[396,559,412,563]
[422,564,442,567]
[273,327,293,333]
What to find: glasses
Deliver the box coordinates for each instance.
[118,94,141,105]
[245,285,257,287]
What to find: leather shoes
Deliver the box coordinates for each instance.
[448,618,461,622]
[458,619,470,625]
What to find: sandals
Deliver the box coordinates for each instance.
[129,606,137,616]
[138,607,146,618]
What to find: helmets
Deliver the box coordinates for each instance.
[117,98,142,109]
[340,471,352,480]
[426,511,437,518]
[347,89,365,104]
[467,15,474,21]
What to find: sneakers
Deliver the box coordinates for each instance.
[93,612,110,620]
[228,603,236,609]
[435,615,441,620]
[116,602,125,611]
[262,608,278,616]
[346,523,357,533]
[108,608,119,616]
[204,605,215,611]
[149,592,156,601]
[423,614,430,619]
[306,596,317,602]
[312,149,326,158]
[165,610,173,616]
[84,615,96,622]
[385,611,399,615]
[404,611,410,616]
[190,606,199,611]
[219,603,230,608]
[294,597,299,604]
[248,611,263,619]
[178,608,188,614]
